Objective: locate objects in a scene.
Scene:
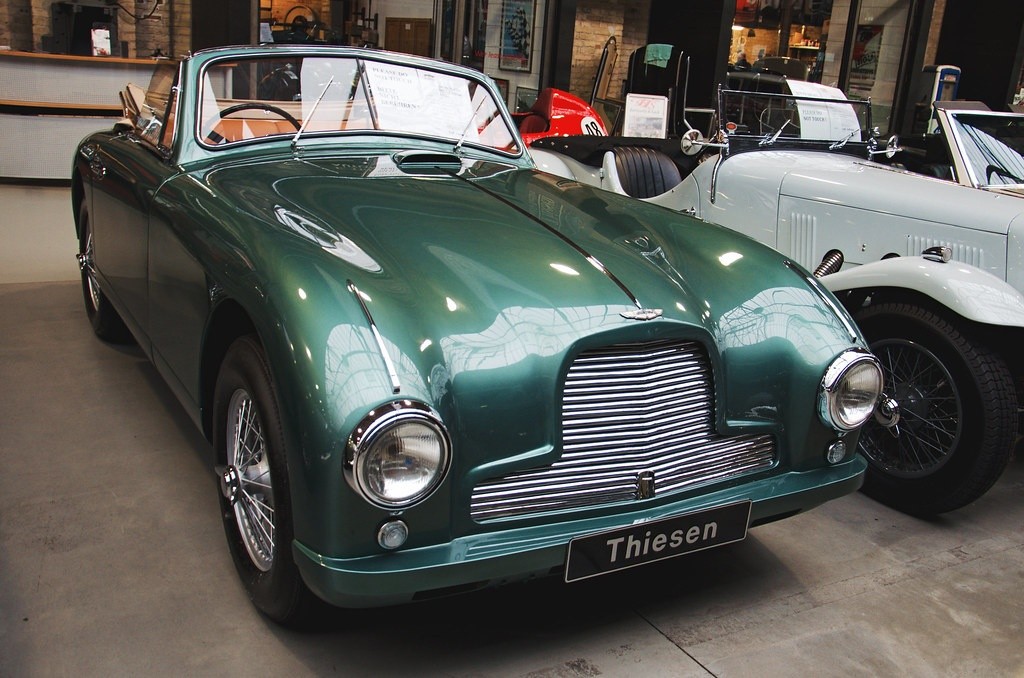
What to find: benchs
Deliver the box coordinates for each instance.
[204,118,370,149]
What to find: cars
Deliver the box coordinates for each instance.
[518,59,1024,521]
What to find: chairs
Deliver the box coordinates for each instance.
[588,35,718,138]
[610,145,682,200]
[921,135,950,179]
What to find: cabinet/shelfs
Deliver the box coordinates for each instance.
[385,16,433,58]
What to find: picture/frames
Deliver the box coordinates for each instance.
[514,86,539,115]
[498,0,537,73]
[489,76,509,109]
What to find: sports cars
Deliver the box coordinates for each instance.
[68,43,892,631]
